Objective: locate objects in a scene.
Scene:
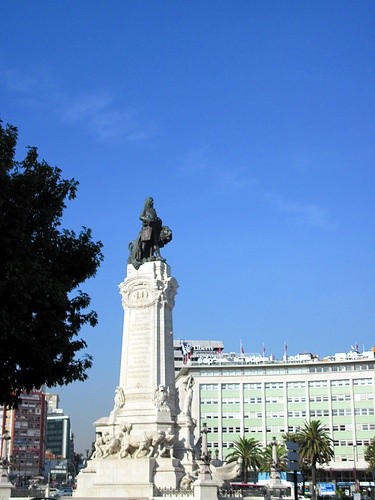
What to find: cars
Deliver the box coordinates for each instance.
[39,478,76,497]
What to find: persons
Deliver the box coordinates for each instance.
[91,425,175,458]
[138,197,167,262]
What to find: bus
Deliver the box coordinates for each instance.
[229,472,375,500]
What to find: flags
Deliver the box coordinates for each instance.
[217,341,222,354]
[262,343,266,353]
[240,341,244,354]
[283,342,288,354]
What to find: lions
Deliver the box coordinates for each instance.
[127,226,173,261]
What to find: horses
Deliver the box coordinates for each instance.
[120,430,166,459]
